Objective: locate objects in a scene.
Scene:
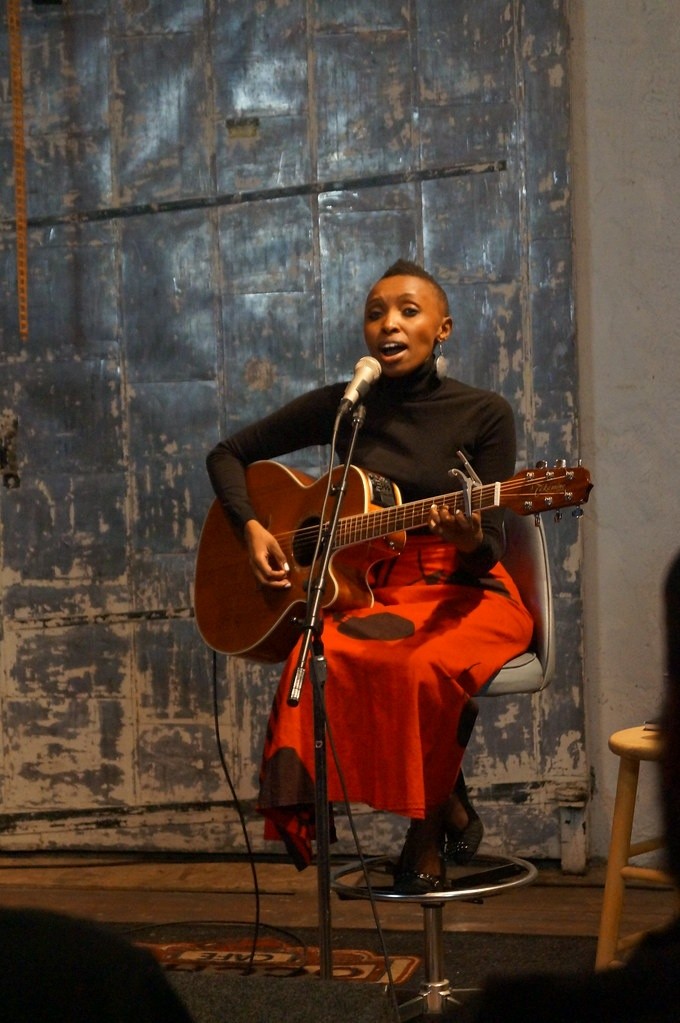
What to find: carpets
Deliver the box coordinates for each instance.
[101,920,599,1023]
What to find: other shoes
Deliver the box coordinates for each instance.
[445,772,484,864]
[394,828,445,893]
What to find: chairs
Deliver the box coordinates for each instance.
[330,508,557,1023]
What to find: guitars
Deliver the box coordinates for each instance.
[193,458,594,666]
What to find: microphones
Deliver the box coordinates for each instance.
[339,356,381,415]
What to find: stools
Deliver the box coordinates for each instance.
[595,726,674,972]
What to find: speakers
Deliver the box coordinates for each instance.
[156,969,399,1023]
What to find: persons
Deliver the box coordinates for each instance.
[206,258,533,895]
